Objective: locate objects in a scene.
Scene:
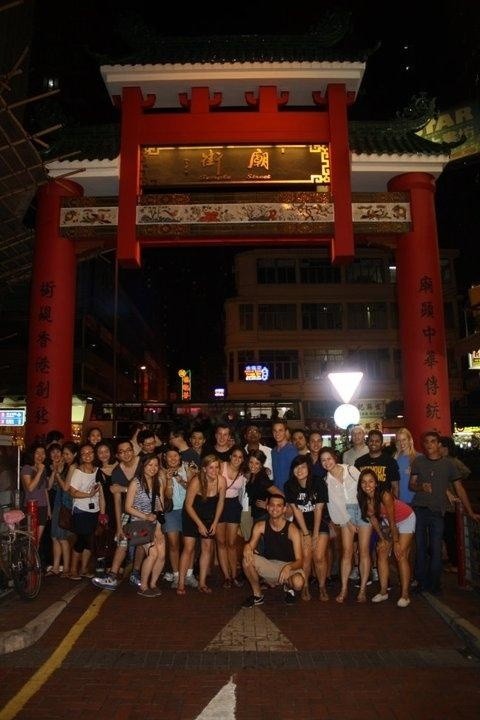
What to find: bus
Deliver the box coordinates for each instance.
[102,398,307,442]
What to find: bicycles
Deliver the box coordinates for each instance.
[0,502,42,602]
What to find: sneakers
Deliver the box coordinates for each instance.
[397,597,410,608]
[128,573,140,587]
[171,576,180,589]
[355,575,374,587]
[370,566,379,581]
[95,557,106,573]
[286,592,297,606]
[348,565,359,581]
[162,571,174,582]
[239,595,265,610]
[91,575,118,591]
[184,575,198,587]
[371,593,389,602]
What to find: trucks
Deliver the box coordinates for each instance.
[85,420,180,440]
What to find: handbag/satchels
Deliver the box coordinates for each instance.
[123,520,156,547]
[58,505,74,532]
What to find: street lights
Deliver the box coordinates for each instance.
[326,369,365,452]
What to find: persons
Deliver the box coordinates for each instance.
[21,403,479,606]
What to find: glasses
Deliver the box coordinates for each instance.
[80,450,94,456]
[117,449,133,455]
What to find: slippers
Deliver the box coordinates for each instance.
[176,586,187,596]
[195,584,213,596]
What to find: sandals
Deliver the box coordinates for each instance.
[317,586,329,602]
[44,570,93,581]
[336,589,349,602]
[300,585,312,602]
[222,576,232,589]
[232,577,245,588]
[357,589,367,602]
[117,573,130,584]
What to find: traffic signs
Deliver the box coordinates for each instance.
[0,419,23,425]
[1,412,23,419]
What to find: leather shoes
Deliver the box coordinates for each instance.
[136,586,156,598]
[412,582,426,597]
[309,576,317,590]
[430,582,442,595]
[325,575,334,591]
[149,585,163,596]
